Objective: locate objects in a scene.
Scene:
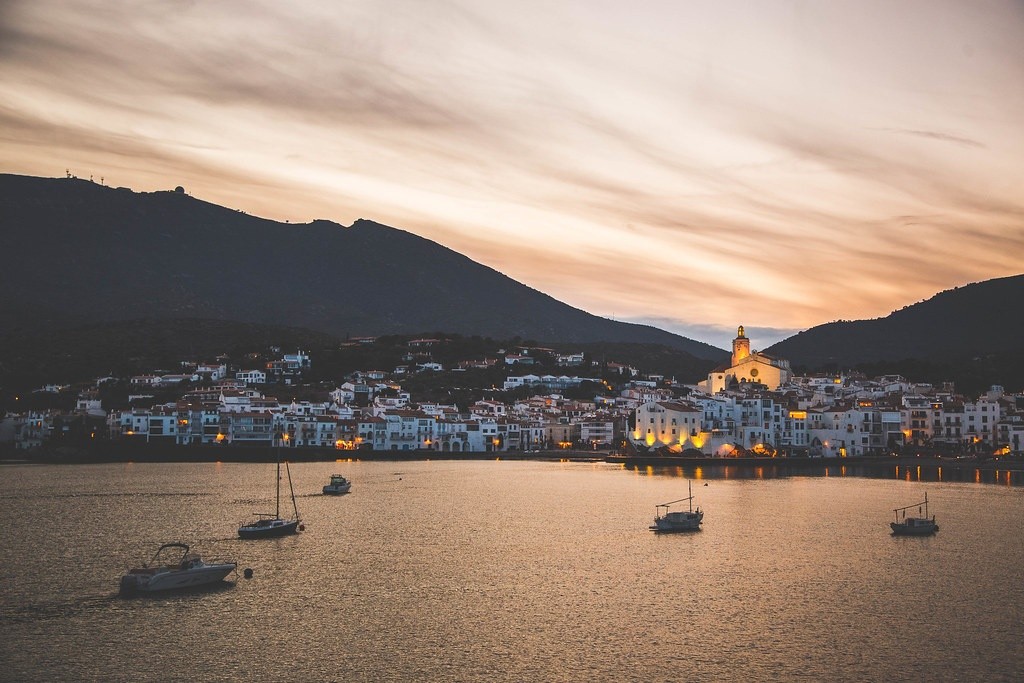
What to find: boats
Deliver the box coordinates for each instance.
[889,492,937,536]
[120,542,236,594]
[648,480,705,531]
[322,474,353,497]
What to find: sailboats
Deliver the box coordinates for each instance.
[238,434,305,539]
